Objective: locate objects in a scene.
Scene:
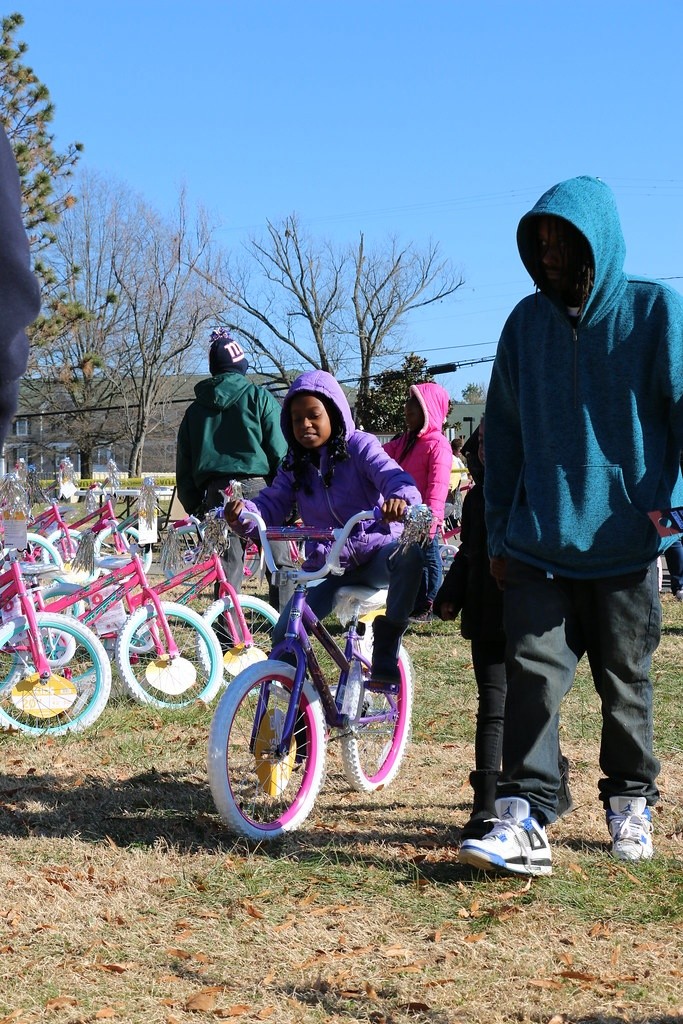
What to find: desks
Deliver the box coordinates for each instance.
[74,489,174,553]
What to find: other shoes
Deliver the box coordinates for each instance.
[409,602,437,622]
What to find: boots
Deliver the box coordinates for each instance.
[294,709,306,763]
[555,756,573,818]
[371,615,409,683]
[461,770,502,840]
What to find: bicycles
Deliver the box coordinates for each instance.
[439,482,477,572]
[1,476,298,738]
[204,495,417,845]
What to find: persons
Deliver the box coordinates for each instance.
[384,384,452,622]
[458,178,683,877]
[176,338,288,656]
[664,541,683,600]
[433,424,573,841]
[224,370,422,763]
[0,125,42,458]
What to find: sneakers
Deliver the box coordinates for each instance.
[459,797,552,877]
[607,796,654,860]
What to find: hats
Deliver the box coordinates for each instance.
[209,336,248,377]
